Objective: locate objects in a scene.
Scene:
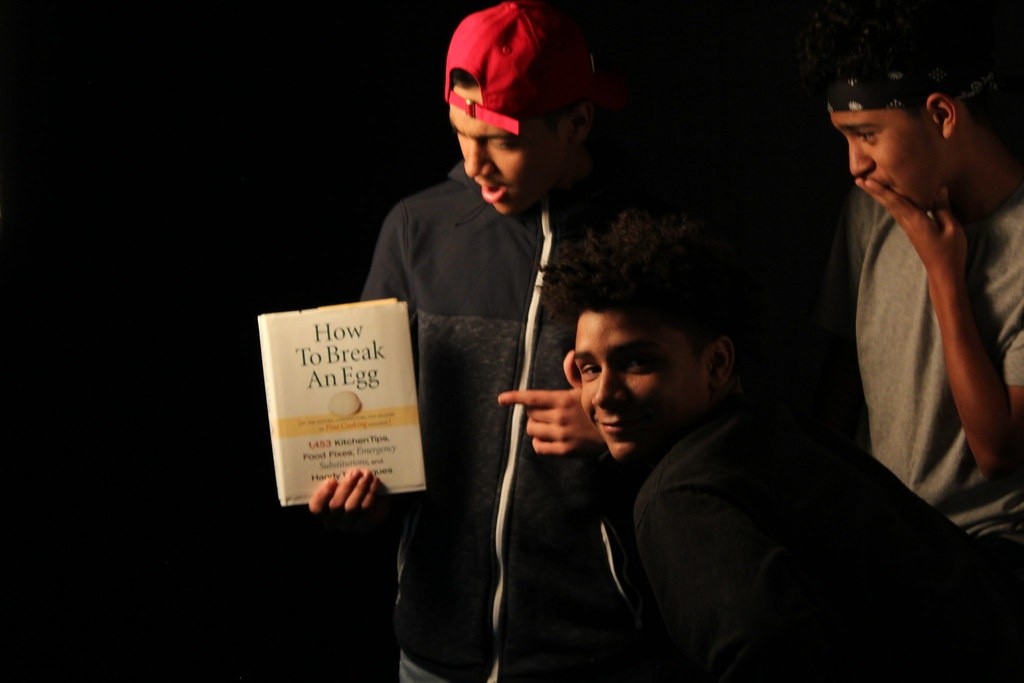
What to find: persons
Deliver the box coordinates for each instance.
[533,210,1023,683]
[786,1,1023,560]
[308,0,661,683]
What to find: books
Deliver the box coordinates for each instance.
[256,298,428,506]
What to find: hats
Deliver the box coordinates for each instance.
[444,0,628,135]
[823,23,1005,111]
